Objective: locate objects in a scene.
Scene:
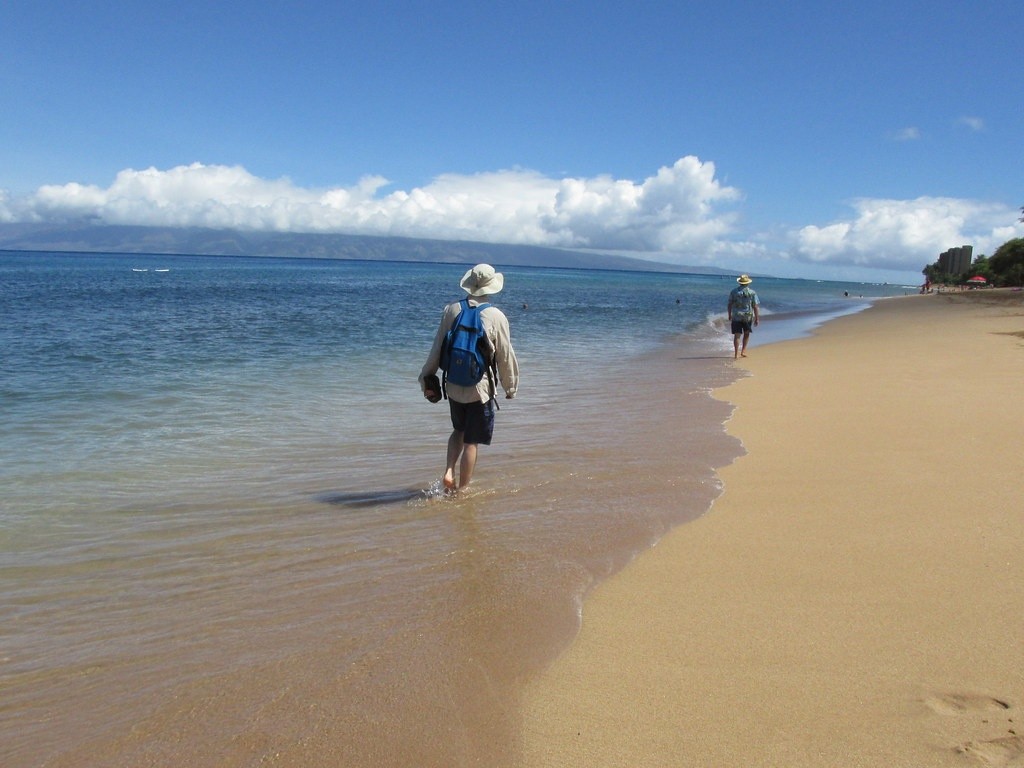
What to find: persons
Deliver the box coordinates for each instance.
[904,291,907,295]
[418,264,520,492]
[843,290,848,296]
[727,274,760,358]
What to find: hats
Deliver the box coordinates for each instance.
[736,274,753,285]
[460,264,504,296]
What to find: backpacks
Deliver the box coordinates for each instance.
[439,299,492,387]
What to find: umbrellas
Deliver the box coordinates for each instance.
[966,276,988,282]
